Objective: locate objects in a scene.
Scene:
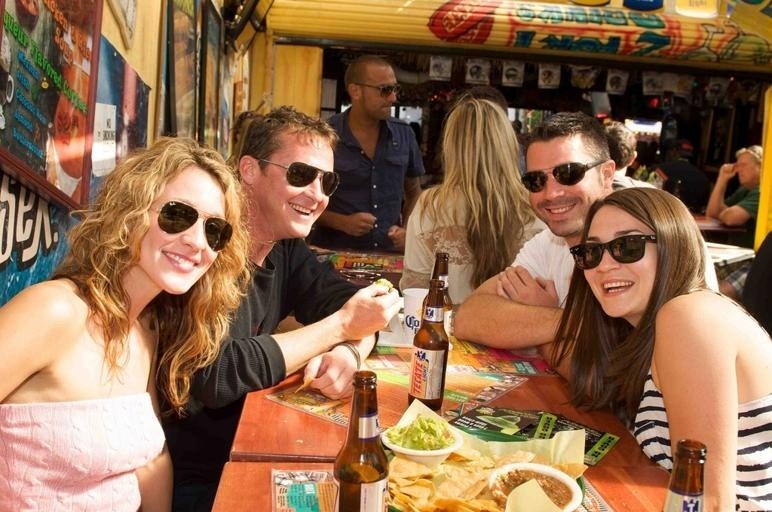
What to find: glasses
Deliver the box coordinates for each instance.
[257,158,340,197]
[149,199,233,252]
[570,235,656,270]
[356,83,401,97]
[521,159,607,192]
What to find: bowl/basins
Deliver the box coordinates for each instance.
[380,425,464,466]
[489,463,583,511]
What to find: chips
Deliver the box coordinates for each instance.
[389,447,588,512]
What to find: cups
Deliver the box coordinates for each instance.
[403,289,428,342]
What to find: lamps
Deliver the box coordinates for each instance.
[591,91,612,119]
[674,0,718,18]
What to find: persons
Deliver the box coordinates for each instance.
[545,186,772,511]
[225,112,261,181]
[145,105,404,507]
[595,120,714,218]
[704,145,763,228]
[447,111,721,390]
[317,54,428,253]
[400,90,540,305]
[510,119,528,178]
[1,139,248,508]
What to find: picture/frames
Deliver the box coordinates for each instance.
[0,0,104,221]
[199,0,222,151]
[165,0,201,137]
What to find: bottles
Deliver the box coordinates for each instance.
[421,253,452,334]
[407,280,448,409]
[331,366,388,512]
[660,438,707,512]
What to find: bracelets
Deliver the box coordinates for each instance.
[340,338,369,373]
